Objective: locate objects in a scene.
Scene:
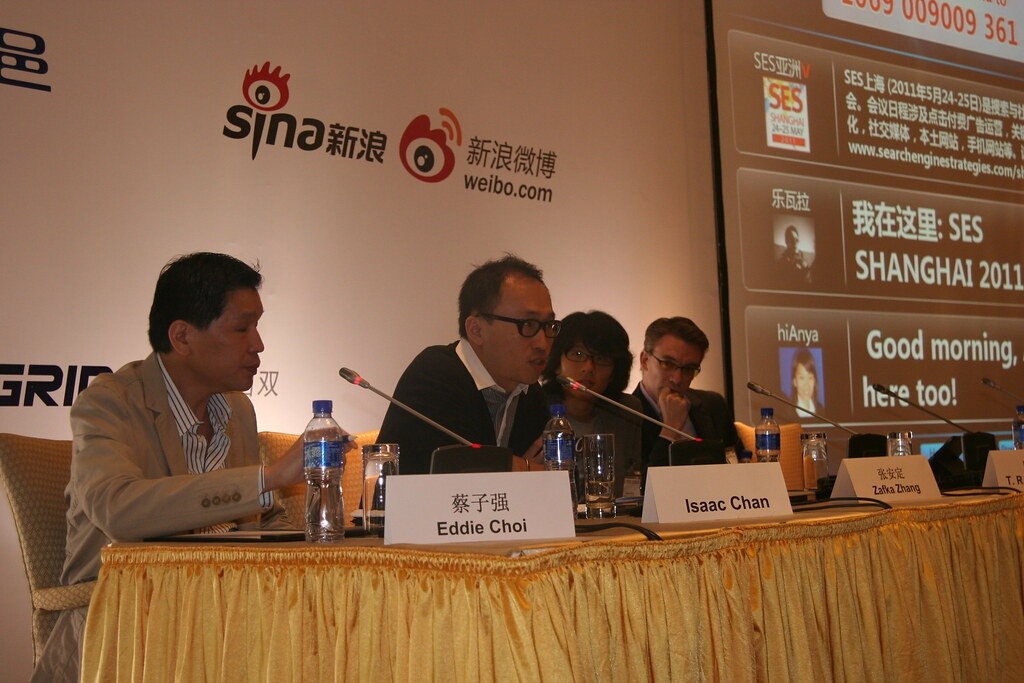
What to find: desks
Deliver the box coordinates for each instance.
[79,487,1024,683]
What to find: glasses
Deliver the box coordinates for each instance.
[475,310,561,338]
[646,349,702,378]
[564,346,615,367]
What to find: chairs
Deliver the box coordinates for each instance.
[736,421,808,489]
[257,430,380,531]
[0,432,97,683]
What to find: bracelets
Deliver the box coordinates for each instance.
[525,459,530,471]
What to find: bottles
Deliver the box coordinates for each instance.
[1012,405,1024,450]
[303,400,344,543]
[543,403,578,521]
[755,408,780,463]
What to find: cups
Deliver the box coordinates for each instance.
[801,432,828,491]
[886,430,912,457]
[362,444,400,539]
[583,434,617,520]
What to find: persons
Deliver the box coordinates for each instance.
[778,345,828,422]
[633,314,749,494]
[512,311,641,506]
[776,228,809,274]
[360,253,559,541]
[30,252,359,683]
